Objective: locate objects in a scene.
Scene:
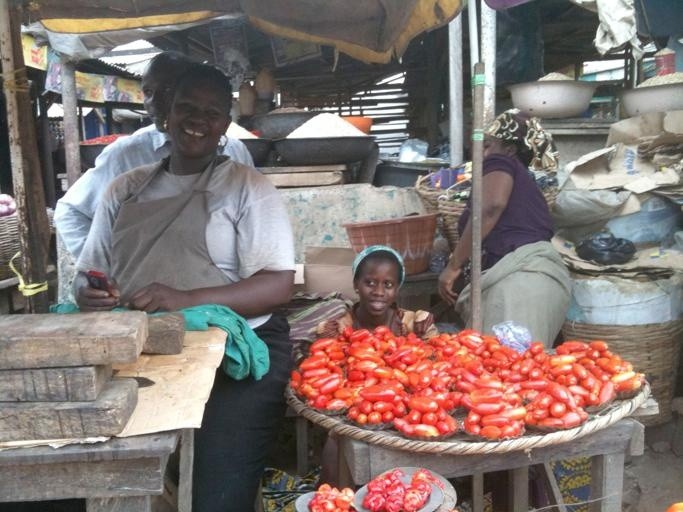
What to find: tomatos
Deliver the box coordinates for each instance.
[290,326,646,440]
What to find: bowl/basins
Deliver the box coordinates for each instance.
[503,80,605,119]
[342,212,441,277]
[618,81,683,117]
[234,109,450,188]
[59,144,109,168]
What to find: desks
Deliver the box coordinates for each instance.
[285,371,662,512]
[0,325,228,511]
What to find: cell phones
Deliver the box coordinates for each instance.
[84,271,115,298]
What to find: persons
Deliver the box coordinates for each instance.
[52,49,256,263]
[71,64,295,512]
[316,243,441,488]
[435,108,573,353]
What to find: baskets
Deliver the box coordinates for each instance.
[0,207,56,280]
[555,312,683,433]
[415,158,557,262]
